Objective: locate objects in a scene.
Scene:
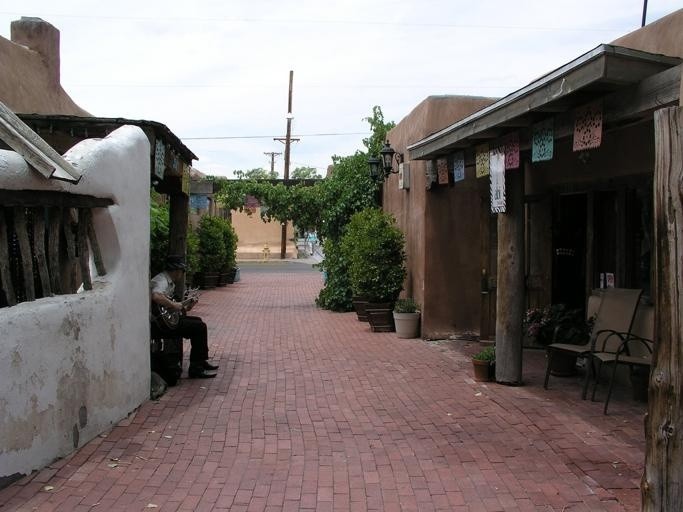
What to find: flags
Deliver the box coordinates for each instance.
[570,98,602,153]
[502,133,520,170]
[435,158,449,185]
[450,149,465,183]
[473,142,490,178]
[529,114,554,164]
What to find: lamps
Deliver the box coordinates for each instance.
[367,139,402,185]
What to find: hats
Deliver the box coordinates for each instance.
[163,254,188,270]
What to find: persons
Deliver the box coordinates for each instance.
[148,253,219,379]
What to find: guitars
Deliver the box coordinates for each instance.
[151,295,199,331]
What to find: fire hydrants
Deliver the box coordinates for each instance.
[262,240,271,263]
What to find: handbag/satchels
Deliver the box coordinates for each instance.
[152,351,183,385]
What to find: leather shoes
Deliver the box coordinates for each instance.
[199,360,219,371]
[188,366,218,378]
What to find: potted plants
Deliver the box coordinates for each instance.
[470,345,496,382]
[339,206,421,339]
[194,212,241,289]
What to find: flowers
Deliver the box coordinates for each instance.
[524,305,552,337]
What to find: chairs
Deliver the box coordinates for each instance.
[543,288,657,415]
